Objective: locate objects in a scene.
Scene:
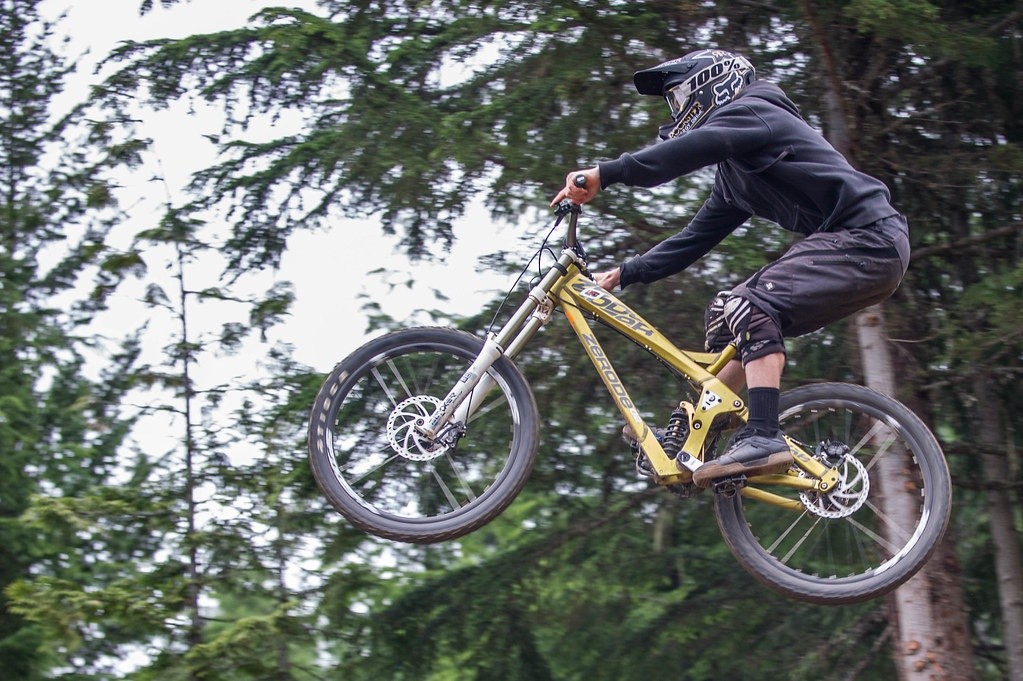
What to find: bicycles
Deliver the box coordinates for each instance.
[306,175,953,607]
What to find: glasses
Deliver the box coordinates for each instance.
[664,85,690,118]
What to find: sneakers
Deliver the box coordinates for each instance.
[692,430,794,487]
[622,425,717,468]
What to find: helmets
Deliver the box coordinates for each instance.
[633,49,754,140]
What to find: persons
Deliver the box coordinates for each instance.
[550,47,909,490]
